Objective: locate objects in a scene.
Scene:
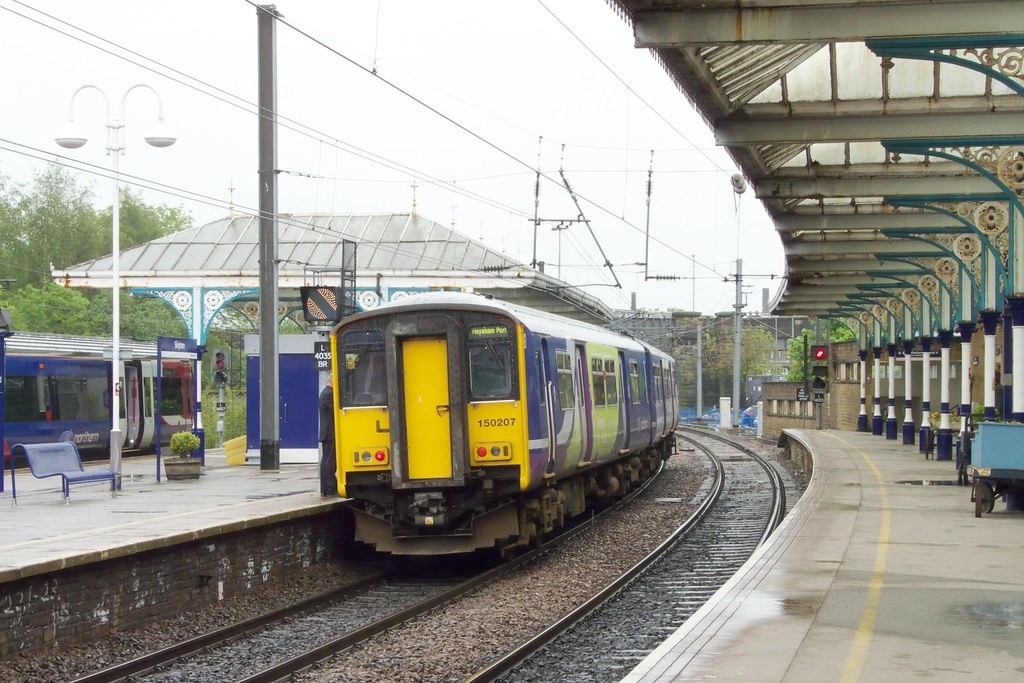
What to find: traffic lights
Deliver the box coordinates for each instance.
[812,346,828,358]
[302,286,341,323]
[212,349,229,385]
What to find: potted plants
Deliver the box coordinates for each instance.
[164,431,202,480]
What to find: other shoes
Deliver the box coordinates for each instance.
[321,494,338,500]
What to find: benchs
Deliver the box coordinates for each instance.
[11,442,115,506]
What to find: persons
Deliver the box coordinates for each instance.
[318,374,337,498]
[995,370,1003,412]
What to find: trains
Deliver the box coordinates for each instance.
[1,331,194,463]
[327,289,682,560]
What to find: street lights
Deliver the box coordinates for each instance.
[54,84,179,490]
